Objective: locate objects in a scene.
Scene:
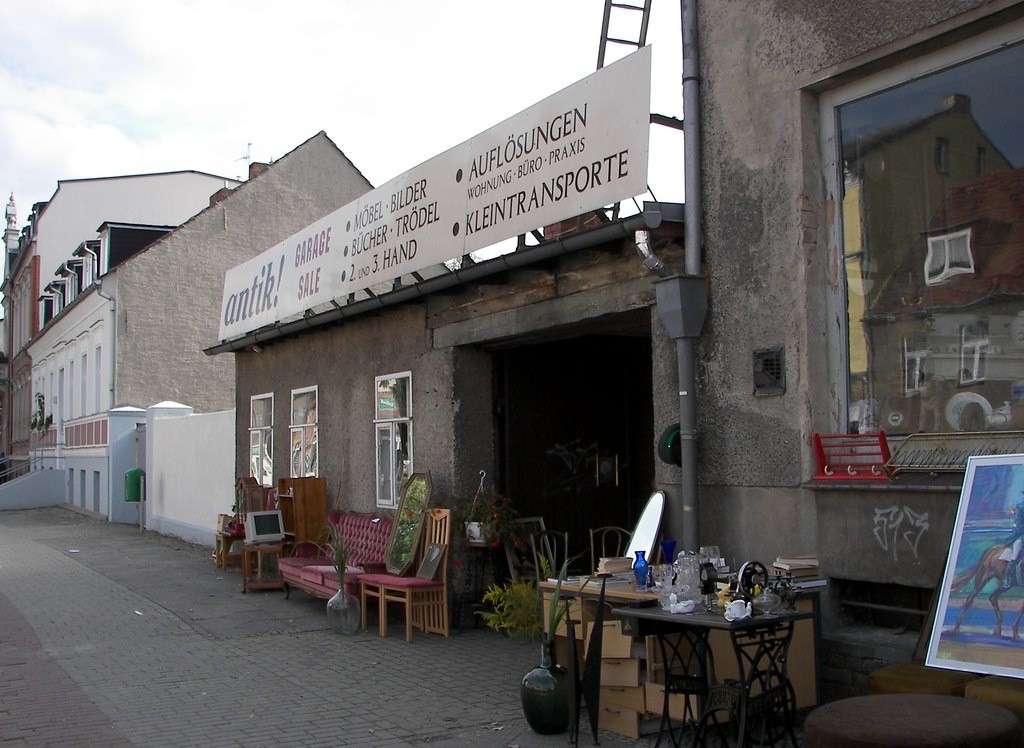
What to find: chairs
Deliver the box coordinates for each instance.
[356,509,451,642]
[589,526,632,573]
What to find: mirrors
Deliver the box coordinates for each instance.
[623,489,666,568]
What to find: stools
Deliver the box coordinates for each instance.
[803,662,1024,748]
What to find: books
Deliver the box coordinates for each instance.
[773,553,828,588]
[594,556,633,577]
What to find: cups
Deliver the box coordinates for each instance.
[652,546,721,612]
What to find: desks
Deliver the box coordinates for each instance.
[244,544,284,593]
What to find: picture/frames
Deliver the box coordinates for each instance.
[924,454,1024,680]
[503,517,555,583]
[386,470,433,576]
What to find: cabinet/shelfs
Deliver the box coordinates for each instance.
[216,477,327,594]
[539,571,822,738]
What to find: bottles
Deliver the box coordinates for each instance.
[633,551,648,589]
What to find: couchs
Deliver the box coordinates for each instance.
[278,511,399,605]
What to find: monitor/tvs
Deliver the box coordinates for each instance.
[244,510,285,546]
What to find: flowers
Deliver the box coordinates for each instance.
[401,485,524,568]
[529,529,569,588]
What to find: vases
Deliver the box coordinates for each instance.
[521,643,572,736]
[463,521,492,543]
[327,586,360,635]
